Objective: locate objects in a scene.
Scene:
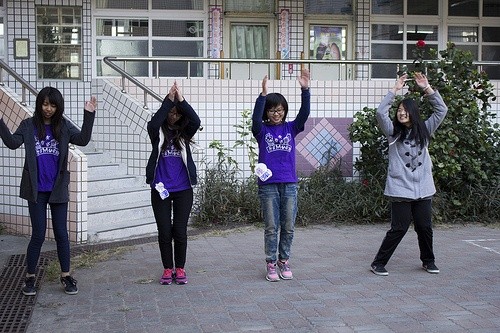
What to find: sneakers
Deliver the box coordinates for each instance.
[21,275,37,295]
[61,275,78,294]
[422,262,440,273]
[370,261,389,276]
[265,262,280,282]
[277,259,293,280]
[175,268,188,285]
[160,268,174,285]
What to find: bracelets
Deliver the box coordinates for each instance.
[422,85,431,91]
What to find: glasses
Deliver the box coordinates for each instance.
[168,111,182,117]
[267,108,285,115]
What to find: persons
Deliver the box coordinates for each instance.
[370,73,448,276]
[146,85,200,285]
[0,87,98,296]
[252,69,311,282]
